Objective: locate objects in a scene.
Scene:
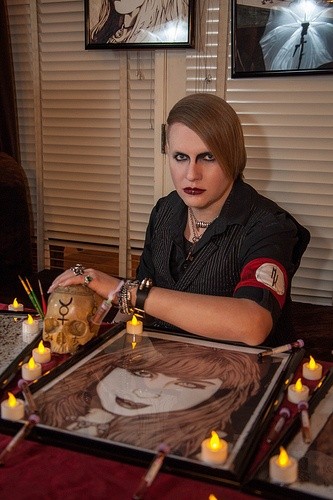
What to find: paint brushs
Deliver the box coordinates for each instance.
[18,274,46,319]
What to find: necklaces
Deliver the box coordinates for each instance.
[189,207,218,243]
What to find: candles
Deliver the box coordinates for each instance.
[269,448,297,484]
[303,354,321,380]
[124,316,142,334]
[1,393,26,421]
[288,377,308,406]
[7,298,28,313]
[21,339,50,381]
[201,433,227,465]
[22,315,38,333]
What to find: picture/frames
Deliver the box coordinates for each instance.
[240,368,333,500]
[84,0,198,50]
[0,321,297,487]
[0,315,46,389]
[229,1,333,78]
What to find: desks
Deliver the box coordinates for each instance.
[0,300,333,500]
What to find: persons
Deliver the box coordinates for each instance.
[47,92,297,346]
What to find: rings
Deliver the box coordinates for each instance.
[82,274,92,287]
[72,263,84,276]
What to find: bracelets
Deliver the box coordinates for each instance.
[117,280,140,315]
[134,278,152,319]
